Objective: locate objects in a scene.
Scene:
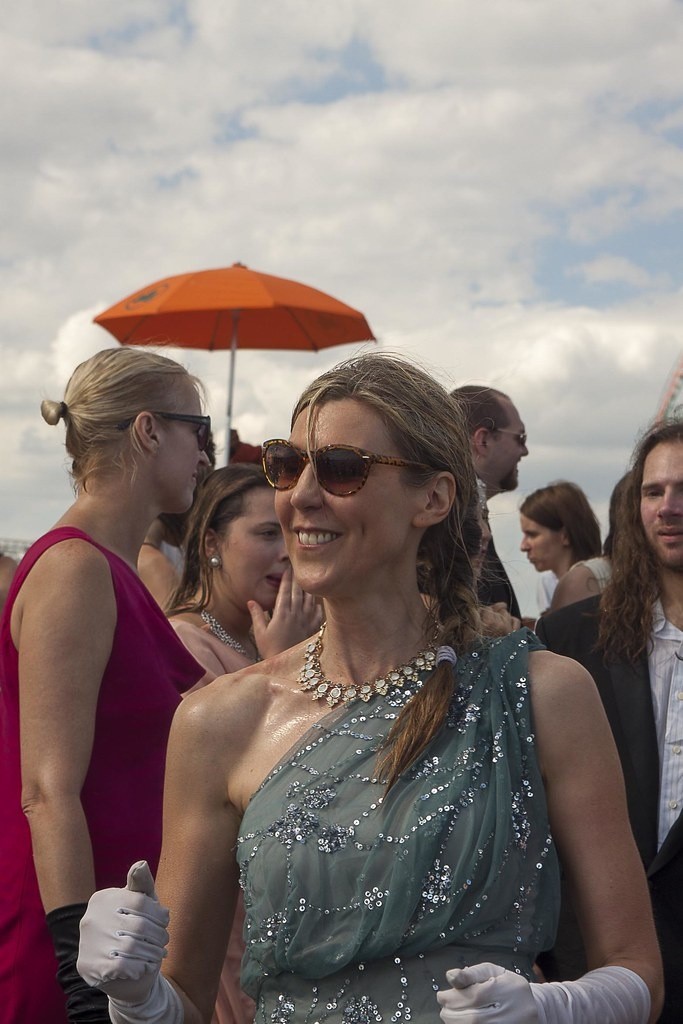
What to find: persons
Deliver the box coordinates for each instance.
[0,354,683,1024]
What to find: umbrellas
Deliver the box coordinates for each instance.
[94,262,377,468]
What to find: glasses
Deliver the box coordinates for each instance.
[261,439,435,499]
[115,410,211,450]
[483,425,527,447]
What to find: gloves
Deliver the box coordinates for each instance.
[437,962,651,1024]
[46,902,112,1024]
[76,859,183,1024]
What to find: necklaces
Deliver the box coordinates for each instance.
[298,622,438,708]
[192,596,262,663]
